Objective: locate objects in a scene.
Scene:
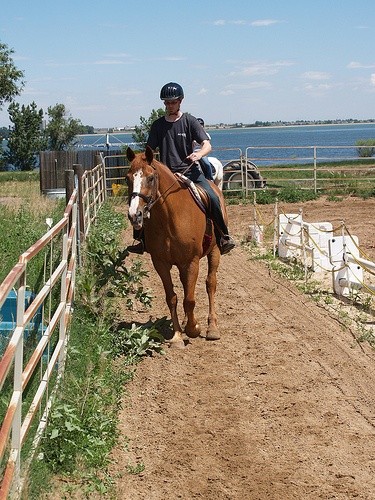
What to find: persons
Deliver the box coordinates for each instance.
[191,117,216,183]
[126,82,234,255]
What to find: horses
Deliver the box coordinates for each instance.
[125,145,228,349]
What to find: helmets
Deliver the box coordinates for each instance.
[160,83,184,100]
[197,118,204,124]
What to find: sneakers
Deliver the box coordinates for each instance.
[218,238,235,254]
[127,243,144,255]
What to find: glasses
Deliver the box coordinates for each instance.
[164,99,181,105]
